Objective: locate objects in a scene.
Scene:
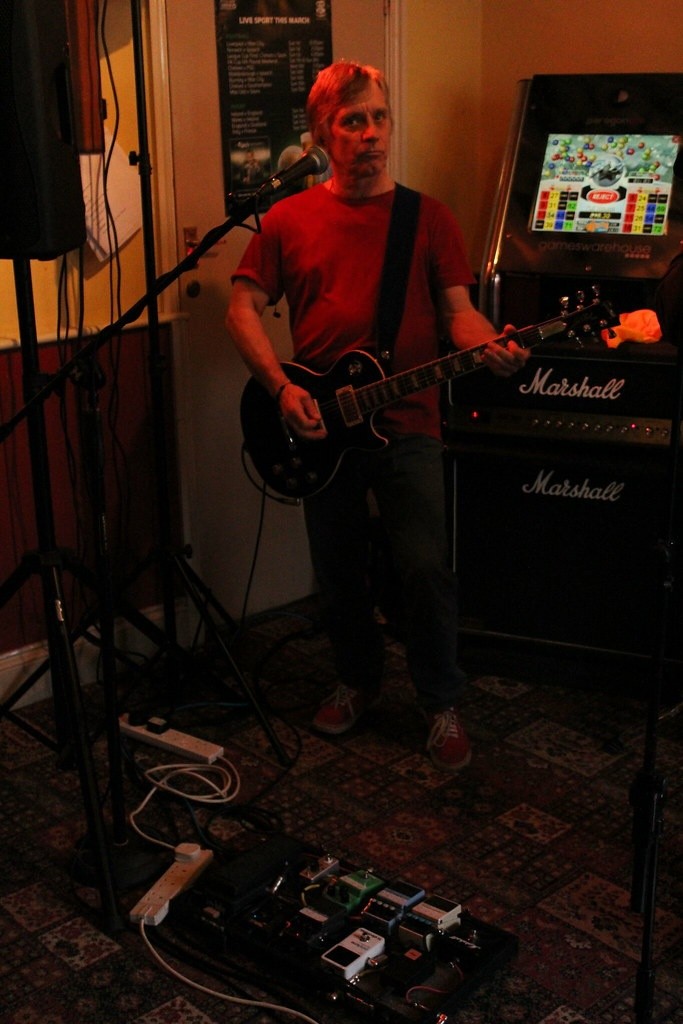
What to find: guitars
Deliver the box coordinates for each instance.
[238,279,621,503]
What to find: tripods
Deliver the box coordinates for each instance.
[0,175,309,940]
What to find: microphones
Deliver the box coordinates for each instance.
[257,145,328,198]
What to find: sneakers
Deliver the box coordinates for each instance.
[314,683,381,732]
[423,706,470,772]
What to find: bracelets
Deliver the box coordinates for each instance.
[276,380,290,401]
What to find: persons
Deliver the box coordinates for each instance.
[224,62,530,771]
[299,132,332,189]
[240,149,263,186]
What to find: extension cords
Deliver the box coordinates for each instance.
[129,850,213,927]
[120,713,224,766]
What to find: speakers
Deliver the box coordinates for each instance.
[0,0,87,261]
[446,445,683,660]
